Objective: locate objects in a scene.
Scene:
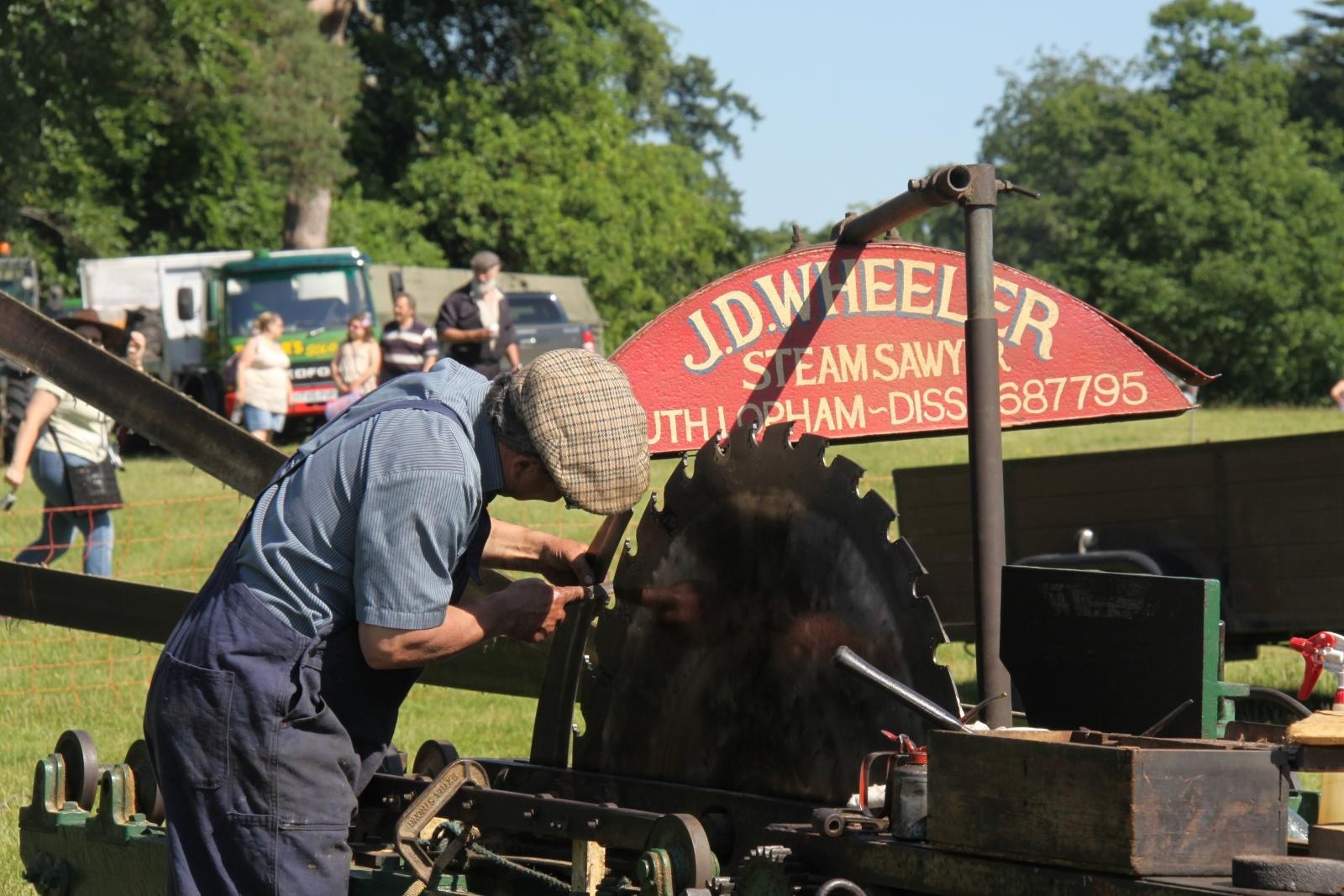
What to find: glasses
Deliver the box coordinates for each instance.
[347,323,364,330]
[541,465,580,512]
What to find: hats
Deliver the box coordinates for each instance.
[469,251,500,273]
[507,347,650,516]
[56,309,124,350]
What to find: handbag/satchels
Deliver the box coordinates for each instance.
[65,456,123,517]
[231,402,244,425]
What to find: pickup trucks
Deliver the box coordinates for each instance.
[498,287,592,369]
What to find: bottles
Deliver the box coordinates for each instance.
[892,752,927,840]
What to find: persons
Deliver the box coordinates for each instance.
[235,312,294,442]
[4,308,146,577]
[435,252,520,380]
[377,293,437,385]
[331,314,381,397]
[144,348,650,896]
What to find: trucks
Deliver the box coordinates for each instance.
[77,245,374,445]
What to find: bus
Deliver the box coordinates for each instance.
[1,239,42,468]
